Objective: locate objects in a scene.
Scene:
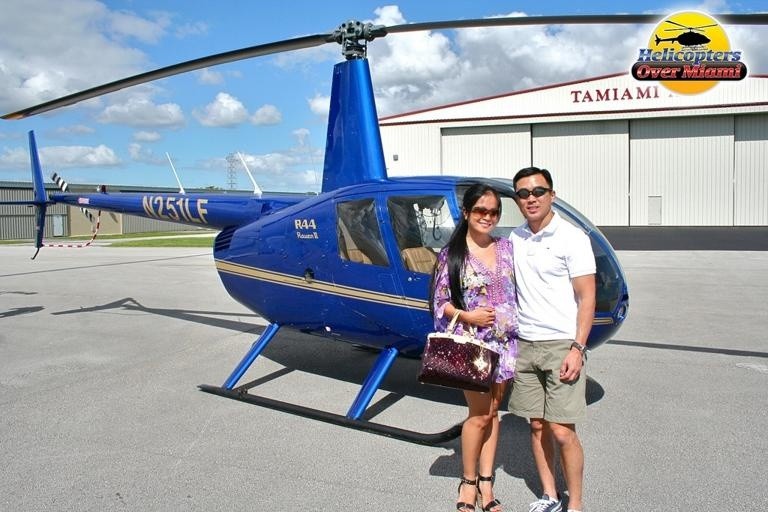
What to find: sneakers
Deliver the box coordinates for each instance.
[527,494,563,512]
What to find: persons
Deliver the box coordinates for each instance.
[507,167,597,512]
[429,184,520,512]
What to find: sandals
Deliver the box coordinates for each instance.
[458,477,477,512]
[478,473,504,512]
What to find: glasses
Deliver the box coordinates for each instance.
[465,205,500,219]
[515,185,552,199]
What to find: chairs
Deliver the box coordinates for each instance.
[345,242,442,277]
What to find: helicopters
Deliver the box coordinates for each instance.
[1,12,768,447]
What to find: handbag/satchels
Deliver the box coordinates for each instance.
[416,310,500,393]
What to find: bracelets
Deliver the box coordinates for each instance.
[569,342,591,361]
